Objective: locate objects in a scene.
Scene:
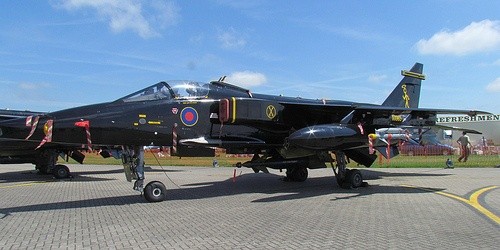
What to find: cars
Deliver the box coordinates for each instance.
[400,137,456,157]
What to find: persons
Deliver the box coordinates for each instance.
[456,131,474,162]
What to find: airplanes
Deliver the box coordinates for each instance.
[0,62,496,203]
